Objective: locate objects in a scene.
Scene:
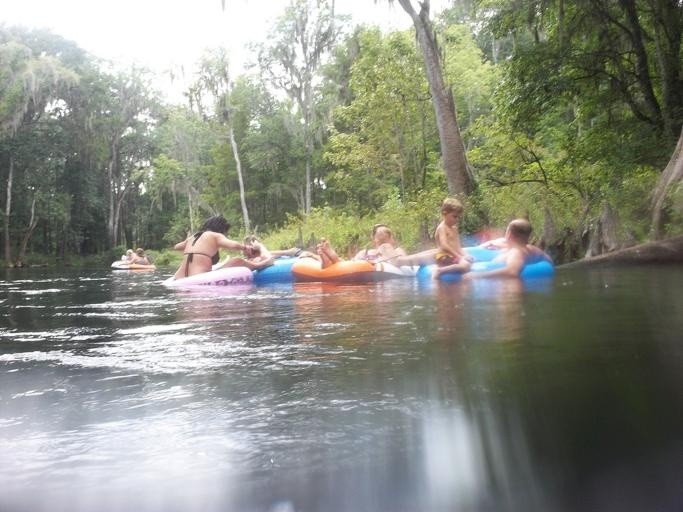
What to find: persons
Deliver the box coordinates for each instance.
[121,249,134,260]
[172,216,274,279]
[118,246,152,267]
[382,219,559,282]
[299,224,406,270]
[374,226,396,264]
[433,197,475,279]
[242,235,302,264]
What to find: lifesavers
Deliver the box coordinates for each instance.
[416,247,552,282]
[163,268,252,287]
[112,261,155,269]
[252,257,291,282]
[291,261,407,282]
